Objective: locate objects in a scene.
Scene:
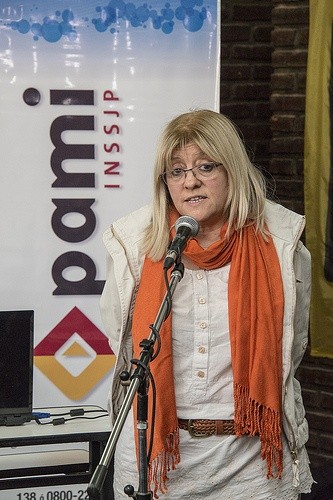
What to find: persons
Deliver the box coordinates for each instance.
[100,110,317,500]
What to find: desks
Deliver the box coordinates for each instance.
[0,405,112,500]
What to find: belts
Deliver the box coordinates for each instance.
[178,419,260,439]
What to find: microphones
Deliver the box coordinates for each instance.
[163,215,201,271]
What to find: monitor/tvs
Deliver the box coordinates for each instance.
[0,310,35,426]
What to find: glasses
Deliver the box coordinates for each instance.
[160,162,223,184]
